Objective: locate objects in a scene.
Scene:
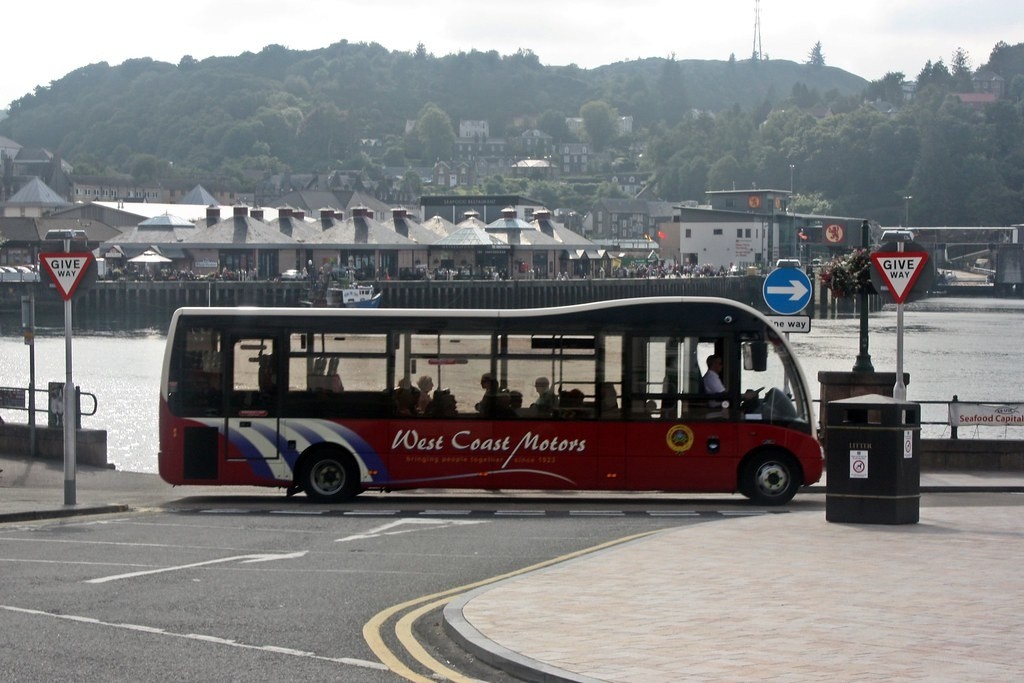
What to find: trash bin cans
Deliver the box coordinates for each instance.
[826,394,921,524]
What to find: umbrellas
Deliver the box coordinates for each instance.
[127,250,173,267]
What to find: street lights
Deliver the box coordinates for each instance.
[44,228,89,507]
[879,230,915,401]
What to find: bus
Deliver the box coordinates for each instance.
[156,293,824,506]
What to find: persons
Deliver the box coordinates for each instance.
[700,354,756,419]
[622,262,734,278]
[416,373,618,422]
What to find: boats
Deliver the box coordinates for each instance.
[297,266,384,308]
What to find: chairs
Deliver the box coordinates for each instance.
[259,354,722,418]
